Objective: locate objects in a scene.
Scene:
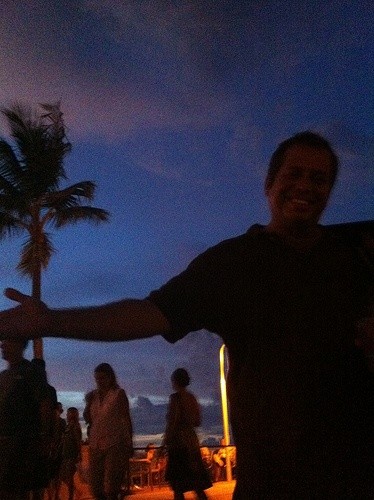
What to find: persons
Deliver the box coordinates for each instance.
[0,337,212,500]
[0,130,374,500]
[156,367,213,500]
